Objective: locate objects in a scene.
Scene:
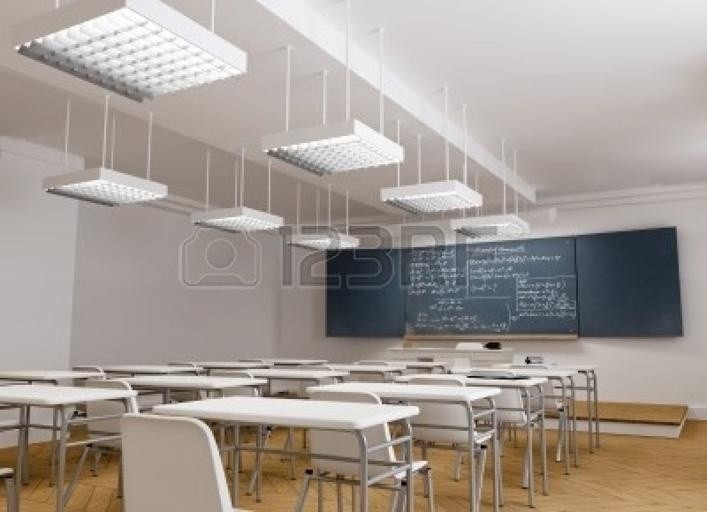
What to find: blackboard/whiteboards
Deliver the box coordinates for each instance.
[325,249,406,339]
[406,235,577,340]
[575,228,683,339]
